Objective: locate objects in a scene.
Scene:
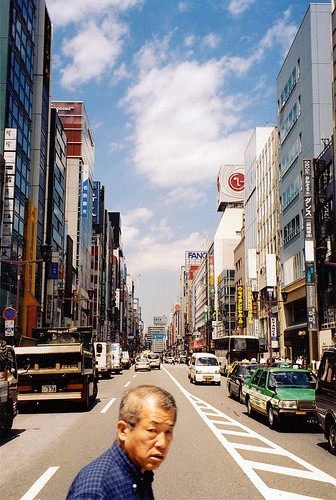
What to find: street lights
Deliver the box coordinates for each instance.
[57,287,96,327]
[251,291,289,367]
[0,241,53,346]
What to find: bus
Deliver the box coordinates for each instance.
[211,334,261,378]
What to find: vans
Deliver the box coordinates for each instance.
[314,344,336,457]
[187,352,222,386]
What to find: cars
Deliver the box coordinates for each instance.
[225,358,267,401]
[239,365,318,430]
[134,349,187,371]
[0,338,20,439]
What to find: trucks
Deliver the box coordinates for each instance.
[121,350,132,370]
[13,325,99,410]
[111,342,123,374]
[93,341,112,379]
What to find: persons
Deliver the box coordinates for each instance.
[66,384,177,500]
[273,356,290,362]
[296,355,306,369]
